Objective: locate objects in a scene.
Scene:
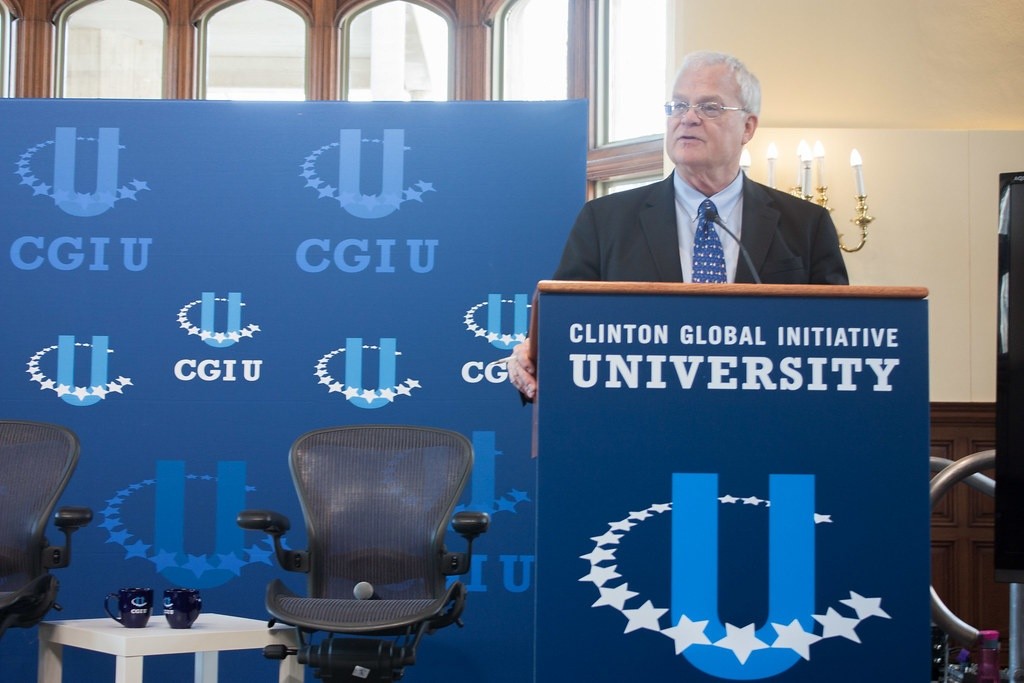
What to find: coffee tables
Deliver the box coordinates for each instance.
[34,611,306,683]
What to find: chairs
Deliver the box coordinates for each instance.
[235,424,490,682]
[0,419,95,640]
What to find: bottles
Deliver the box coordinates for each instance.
[977,630,1000,683]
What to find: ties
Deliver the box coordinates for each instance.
[692,199,727,284]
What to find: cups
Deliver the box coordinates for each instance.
[103,585,154,628]
[163,588,203,628]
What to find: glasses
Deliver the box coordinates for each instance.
[664,100,744,119]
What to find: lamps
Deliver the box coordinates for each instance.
[739,137,875,254]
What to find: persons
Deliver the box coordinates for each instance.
[507,52,850,407]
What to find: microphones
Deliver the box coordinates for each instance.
[353,581,383,601]
[705,209,761,285]
